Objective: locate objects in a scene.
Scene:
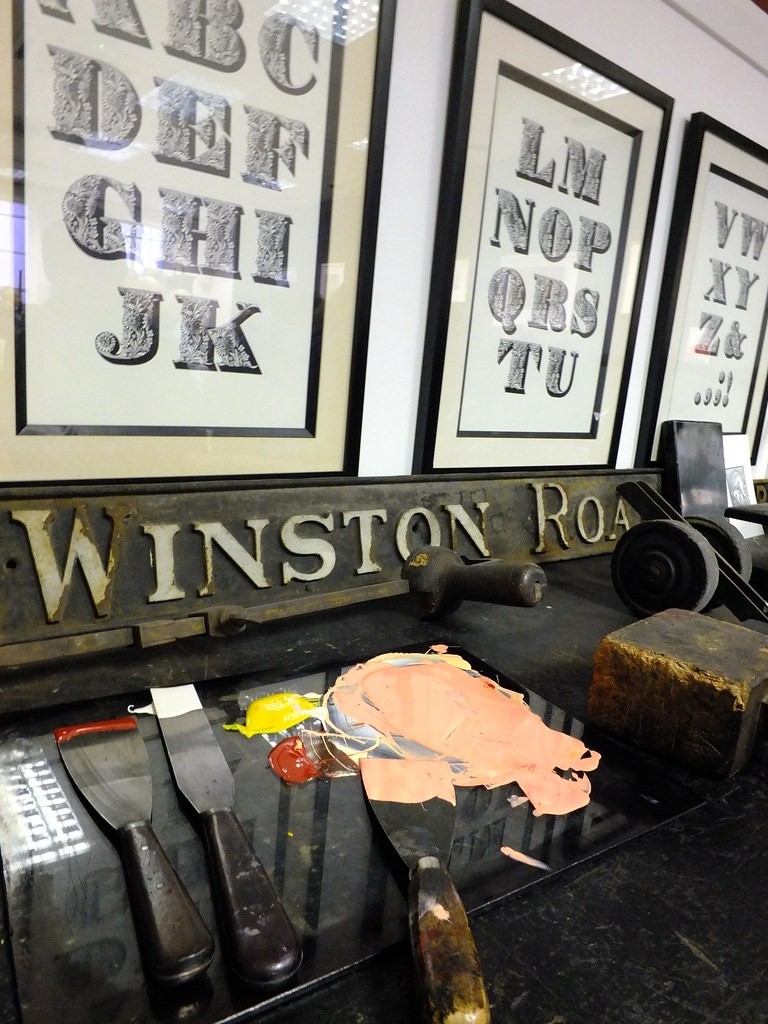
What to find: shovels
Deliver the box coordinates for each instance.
[359,758,491,1024]
[150,683,304,995]
[52,716,216,994]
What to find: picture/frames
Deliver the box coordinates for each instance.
[633,110,768,469]
[409,0,677,475]
[0,0,398,489]
